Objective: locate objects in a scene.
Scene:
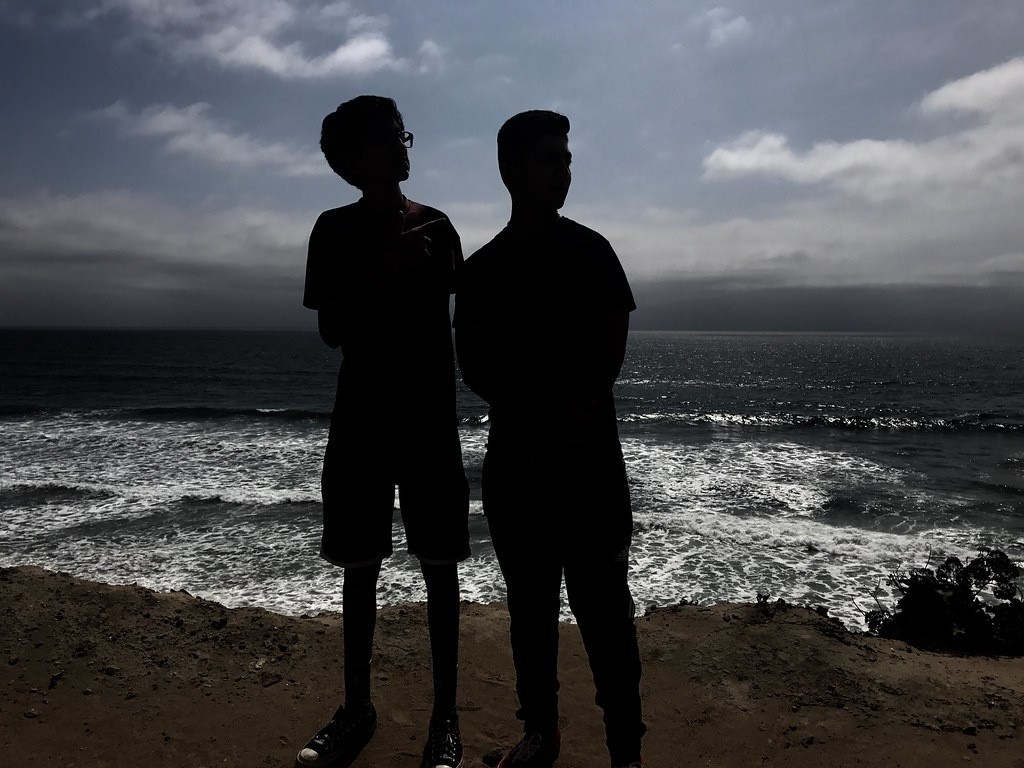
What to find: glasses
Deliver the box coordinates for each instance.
[348,129,413,150]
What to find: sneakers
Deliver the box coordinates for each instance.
[296,698,376,767]
[426,710,464,767]
[496,725,561,767]
[611,750,640,766]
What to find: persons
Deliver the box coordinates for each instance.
[452,108,648,768]
[294,94,470,768]
